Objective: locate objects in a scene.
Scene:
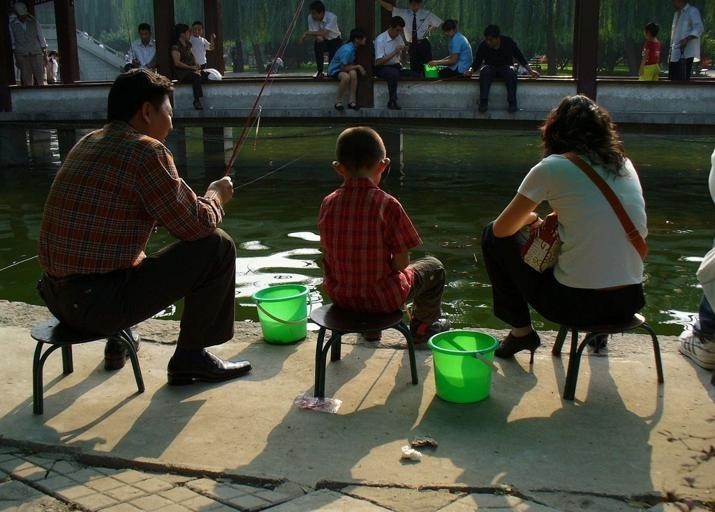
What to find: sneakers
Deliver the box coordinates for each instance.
[676,324,715,372]
[335,102,345,110]
[410,316,451,344]
[348,102,361,110]
[362,332,381,342]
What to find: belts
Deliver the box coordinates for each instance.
[42,269,93,288]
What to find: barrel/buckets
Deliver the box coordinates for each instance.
[423,63,440,80]
[427,330,506,404]
[250,283,312,346]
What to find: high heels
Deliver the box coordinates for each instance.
[493,327,541,366]
[584,332,608,354]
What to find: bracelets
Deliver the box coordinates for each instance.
[528,213,542,227]
[146,64,151,69]
[43,48,48,53]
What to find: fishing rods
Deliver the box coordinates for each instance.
[405,2,440,48]
[223,1,304,180]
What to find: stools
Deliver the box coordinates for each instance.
[552,312,665,400]
[30,317,146,415]
[308,303,420,402]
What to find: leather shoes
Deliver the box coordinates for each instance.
[313,71,325,79]
[104,331,141,369]
[387,101,402,111]
[479,101,488,113]
[168,350,253,385]
[508,104,517,113]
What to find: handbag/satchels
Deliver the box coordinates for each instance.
[518,211,564,272]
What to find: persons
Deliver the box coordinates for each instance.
[8,2,49,85]
[317,126,450,345]
[326,27,367,110]
[667,0,704,80]
[481,90,650,364]
[46,49,59,83]
[38,66,251,385]
[376,0,444,72]
[170,22,208,110]
[427,19,474,78]
[374,16,423,109]
[462,23,540,112]
[297,0,343,79]
[677,146,715,371]
[188,20,217,68]
[638,21,662,80]
[120,22,158,73]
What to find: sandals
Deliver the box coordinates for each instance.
[192,98,205,110]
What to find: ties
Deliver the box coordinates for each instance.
[412,14,417,58]
